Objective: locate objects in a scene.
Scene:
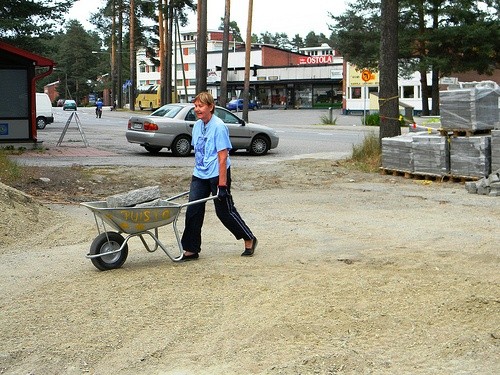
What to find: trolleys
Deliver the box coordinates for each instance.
[79,184,218,271]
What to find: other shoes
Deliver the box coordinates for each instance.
[175,251,199,261]
[241,237,258,256]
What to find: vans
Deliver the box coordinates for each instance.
[36,93,54,129]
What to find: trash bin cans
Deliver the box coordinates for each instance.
[405,106,414,117]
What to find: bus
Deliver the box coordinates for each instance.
[135,84,178,110]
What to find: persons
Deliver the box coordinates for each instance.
[95,98,103,118]
[172,92,259,261]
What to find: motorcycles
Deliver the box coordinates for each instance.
[94,104,102,118]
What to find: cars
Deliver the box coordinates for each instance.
[126,103,279,157]
[56,99,77,111]
[226,99,258,111]
